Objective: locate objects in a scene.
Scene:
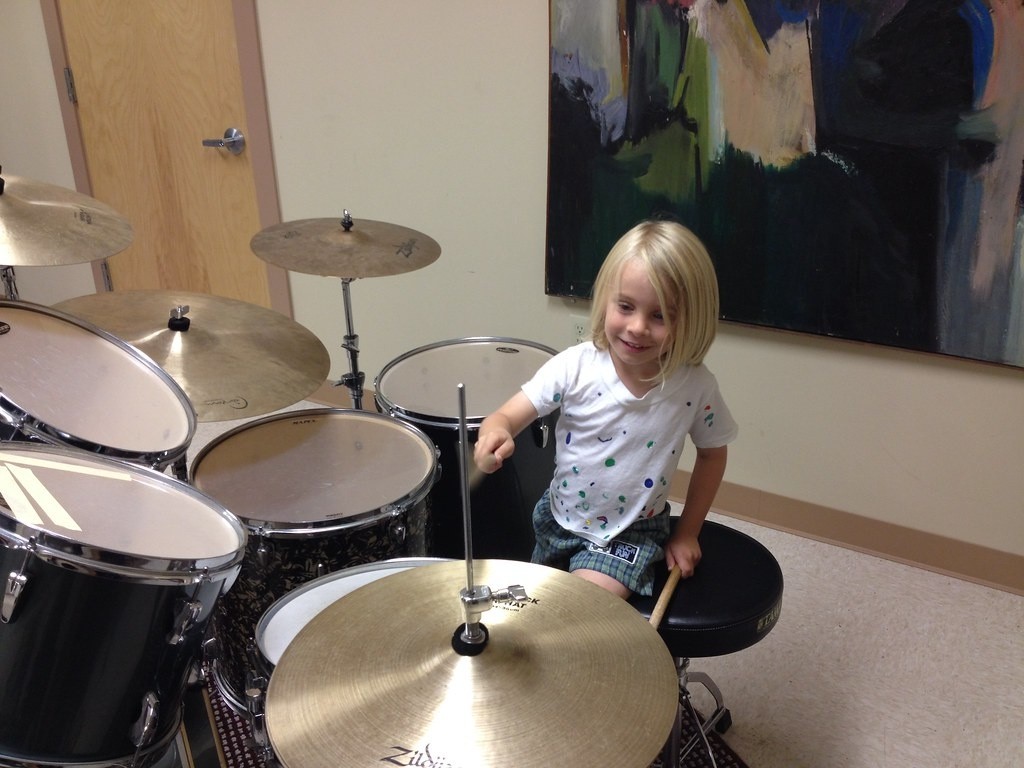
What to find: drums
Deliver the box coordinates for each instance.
[373,334,565,569]
[248,559,462,768]
[188,404,442,720]
[0,442,248,768]
[0,302,198,474]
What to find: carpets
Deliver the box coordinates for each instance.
[199,622,751,768]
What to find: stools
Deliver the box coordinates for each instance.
[630,516,784,768]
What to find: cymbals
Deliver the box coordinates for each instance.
[248,215,442,279]
[263,558,681,768]
[0,173,136,266]
[43,289,331,422]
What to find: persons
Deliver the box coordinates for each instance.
[473,220,740,598]
